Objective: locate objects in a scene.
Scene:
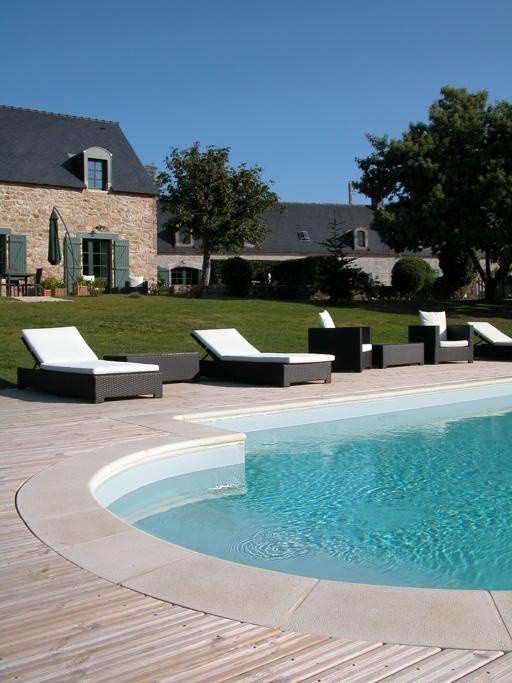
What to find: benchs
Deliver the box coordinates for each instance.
[373,340,426,371]
[106,349,202,389]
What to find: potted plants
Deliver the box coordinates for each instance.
[73,275,93,295]
[41,275,68,298]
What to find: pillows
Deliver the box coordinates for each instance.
[416,309,449,341]
[317,309,337,327]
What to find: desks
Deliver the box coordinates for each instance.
[1,270,38,298]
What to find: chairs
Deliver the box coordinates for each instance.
[306,322,374,372]
[407,322,475,365]
[189,326,339,386]
[5,268,21,297]
[22,267,43,295]
[467,320,511,361]
[20,324,164,404]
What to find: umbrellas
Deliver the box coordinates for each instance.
[48,207,74,294]
